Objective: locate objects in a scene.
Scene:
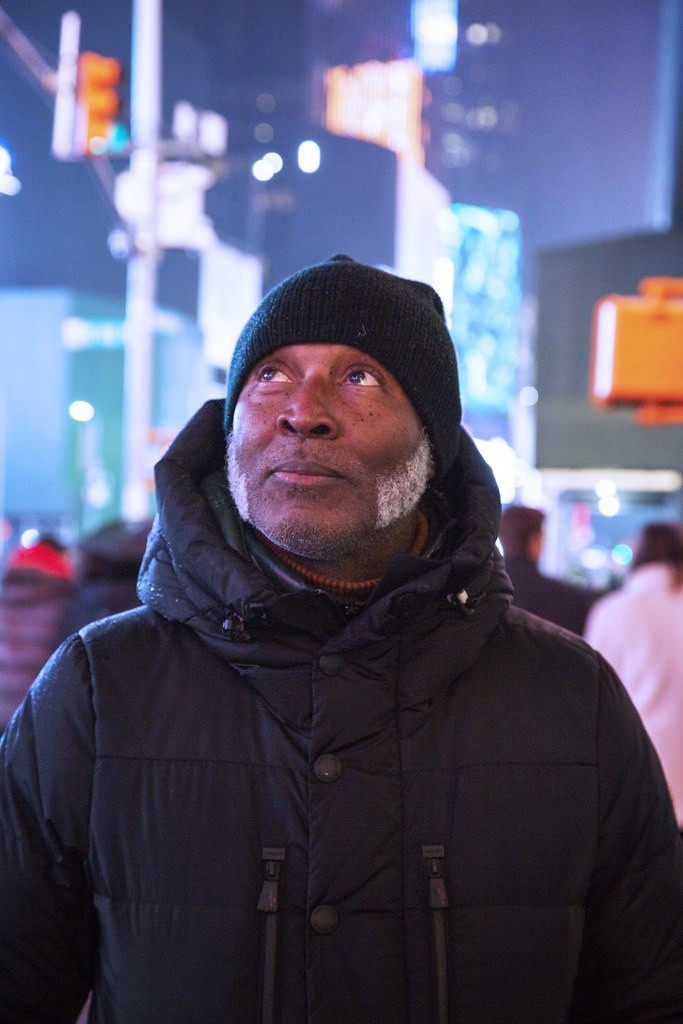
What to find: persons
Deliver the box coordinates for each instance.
[495,505,603,635]
[0,252,682,1024]
[583,520,683,828]
[51,516,154,646]
[0,528,77,733]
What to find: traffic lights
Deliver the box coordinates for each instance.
[72,53,124,159]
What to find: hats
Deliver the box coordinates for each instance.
[221,253,464,509]
